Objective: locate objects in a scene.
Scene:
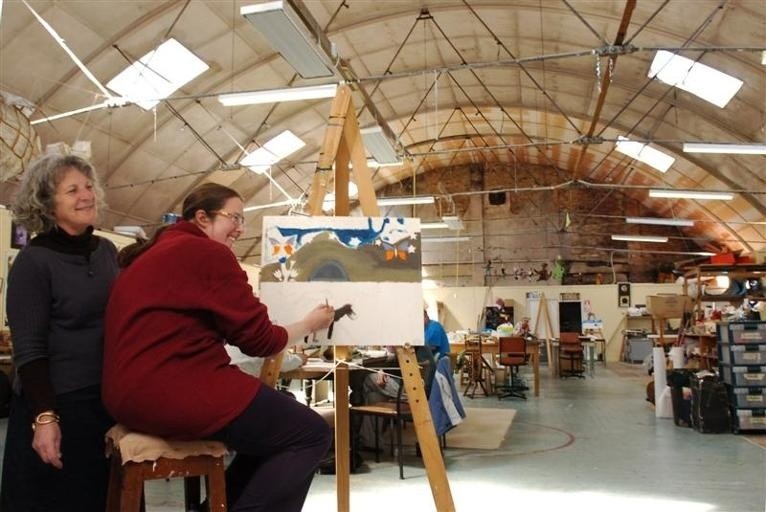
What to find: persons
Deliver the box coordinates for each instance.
[101,183,336,512]
[223,342,308,379]
[0,152,119,512]
[351,309,451,454]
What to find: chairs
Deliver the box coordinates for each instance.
[350,352,458,479]
[559,330,586,381]
[494,336,528,399]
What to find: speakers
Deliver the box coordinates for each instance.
[618,282,631,308]
[489,190,505,205]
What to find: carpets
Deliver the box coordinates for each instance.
[741,430,766,450]
[381,406,517,449]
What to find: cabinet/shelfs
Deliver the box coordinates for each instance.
[620,312,684,363]
[678,261,766,370]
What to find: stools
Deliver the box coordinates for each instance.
[100,425,228,512]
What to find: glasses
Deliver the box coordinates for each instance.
[206,208,245,225]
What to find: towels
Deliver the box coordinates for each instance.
[428,355,466,435]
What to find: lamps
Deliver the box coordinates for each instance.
[240,1,335,81]
[440,213,466,232]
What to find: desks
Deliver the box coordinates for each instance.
[447,341,541,397]
[273,350,401,412]
[549,336,607,368]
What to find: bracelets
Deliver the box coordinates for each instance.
[32,412,60,431]
